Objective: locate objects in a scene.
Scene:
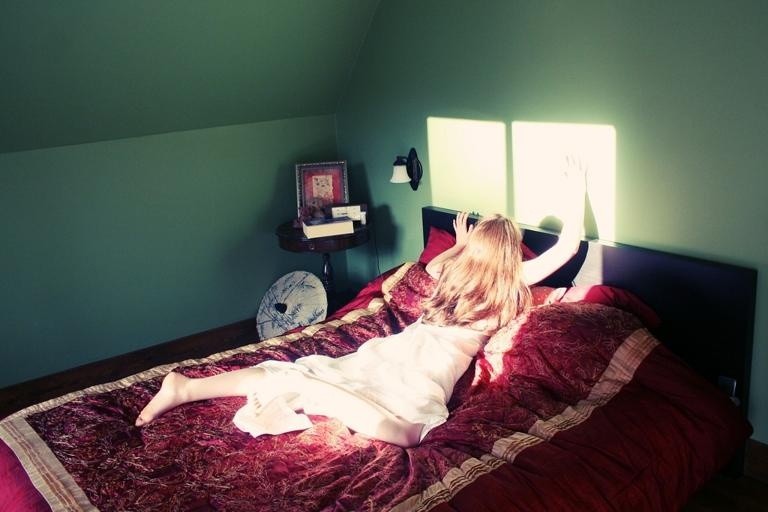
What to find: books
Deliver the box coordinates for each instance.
[300,216,355,240]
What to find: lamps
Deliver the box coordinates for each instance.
[390,148,423,191]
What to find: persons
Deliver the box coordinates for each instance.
[134,153,588,450]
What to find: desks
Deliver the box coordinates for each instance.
[275,220,374,317]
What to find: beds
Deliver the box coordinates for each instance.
[2,206,758,512]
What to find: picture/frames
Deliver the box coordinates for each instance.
[296,160,349,221]
